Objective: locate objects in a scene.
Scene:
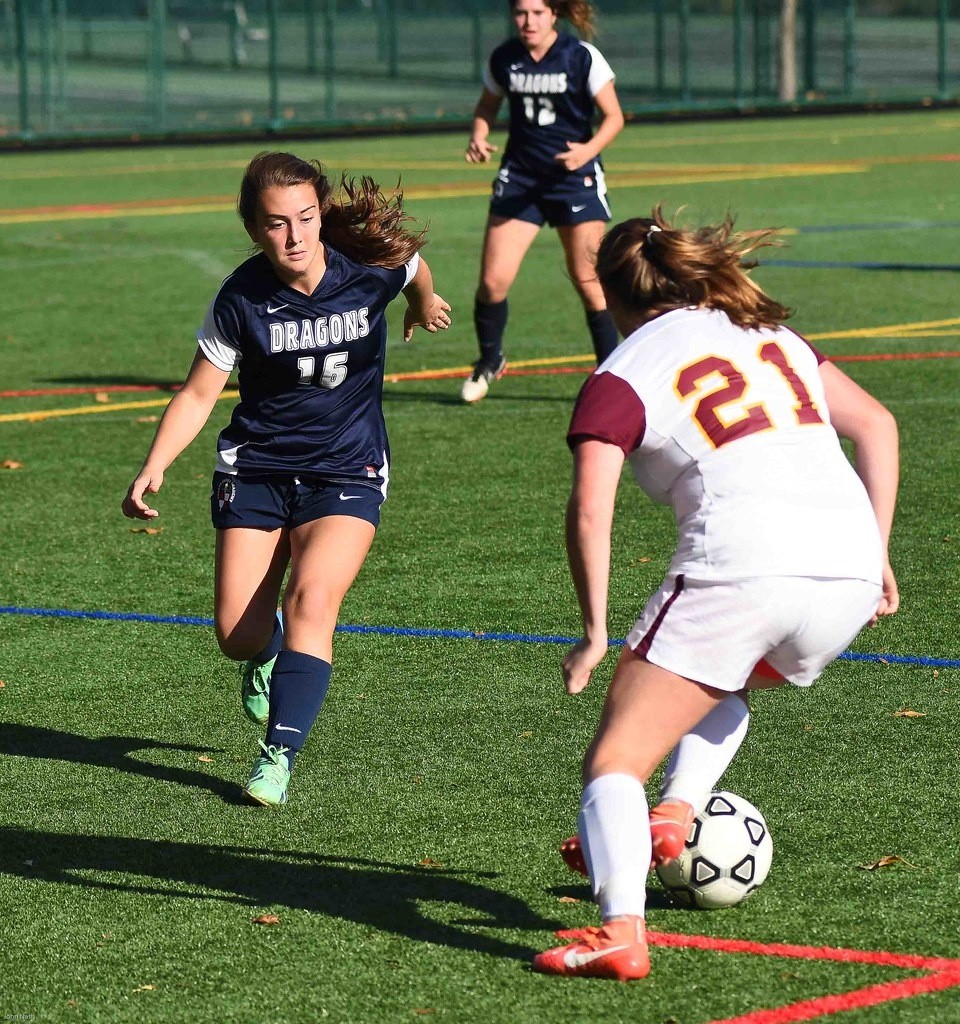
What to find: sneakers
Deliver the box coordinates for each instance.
[461,350,508,403]
[239,607,283,724]
[559,800,695,876]
[239,738,292,810]
[534,914,651,983]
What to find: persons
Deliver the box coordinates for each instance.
[122,152,451,805]
[460,0,623,405]
[533,204,901,979]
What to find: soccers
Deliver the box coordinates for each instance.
[656,791,774,909]
